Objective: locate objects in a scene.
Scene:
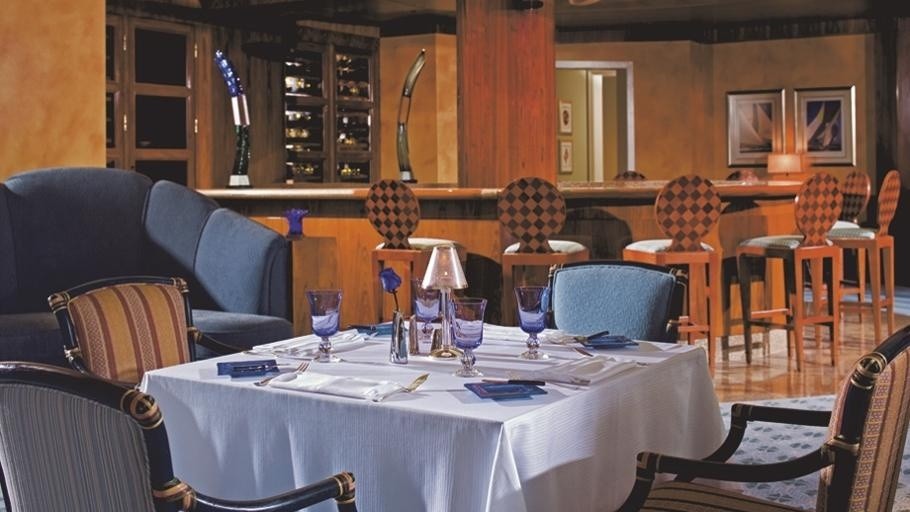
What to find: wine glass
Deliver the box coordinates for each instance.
[449,296,487,375]
[413,281,441,344]
[304,287,343,363]
[515,286,551,360]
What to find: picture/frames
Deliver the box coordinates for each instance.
[788,85,861,166]
[723,89,788,168]
[558,140,575,176]
[557,97,573,135]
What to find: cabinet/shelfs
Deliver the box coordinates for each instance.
[281,41,379,186]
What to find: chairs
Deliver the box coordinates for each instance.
[610,318,908,511]
[541,259,685,351]
[1,352,365,512]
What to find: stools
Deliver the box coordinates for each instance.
[724,167,844,375]
[497,175,591,325]
[804,167,906,352]
[612,166,724,377]
[362,177,468,328]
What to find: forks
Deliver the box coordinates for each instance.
[373,372,434,404]
[254,361,309,387]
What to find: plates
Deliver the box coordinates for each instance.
[576,334,639,348]
[464,380,548,399]
[217,358,280,376]
[353,324,405,338]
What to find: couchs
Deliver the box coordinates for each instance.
[1,161,299,378]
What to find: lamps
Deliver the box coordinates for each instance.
[768,154,806,177]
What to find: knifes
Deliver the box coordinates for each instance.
[482,377,546,386]
[574,330,609,344]
[231,361,290,370]
[346,320,392,332]
